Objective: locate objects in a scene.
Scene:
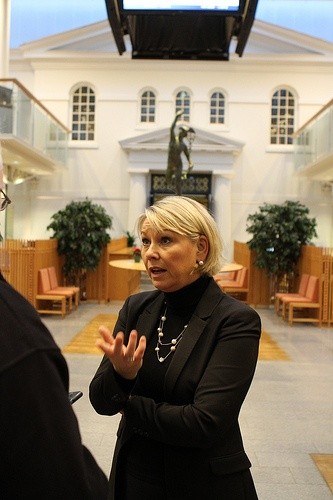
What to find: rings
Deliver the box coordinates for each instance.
[123,355,134,364]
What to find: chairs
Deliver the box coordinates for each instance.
[281,275,322,326]
[212,263,250,305]
[37,269,73,319]
[48,266,80,310]
[276,273,310,317]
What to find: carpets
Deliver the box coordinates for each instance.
[62,312,288,361]
[310,452,333,494]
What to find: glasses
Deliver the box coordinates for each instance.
[0,188,11,211]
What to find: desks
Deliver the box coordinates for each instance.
[109,259,243,273]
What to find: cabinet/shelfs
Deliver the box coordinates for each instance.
[109,248,141,302]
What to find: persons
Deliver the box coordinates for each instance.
[165,110,194,196]
[88,196,261,500]
[0,152,110,500]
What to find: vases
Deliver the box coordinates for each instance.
[134,256,141,262]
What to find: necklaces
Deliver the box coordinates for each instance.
[155,296,191,363]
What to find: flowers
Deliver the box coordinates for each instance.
[132,248,141,256]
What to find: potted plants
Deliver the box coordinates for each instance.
[247,201,318,293]
